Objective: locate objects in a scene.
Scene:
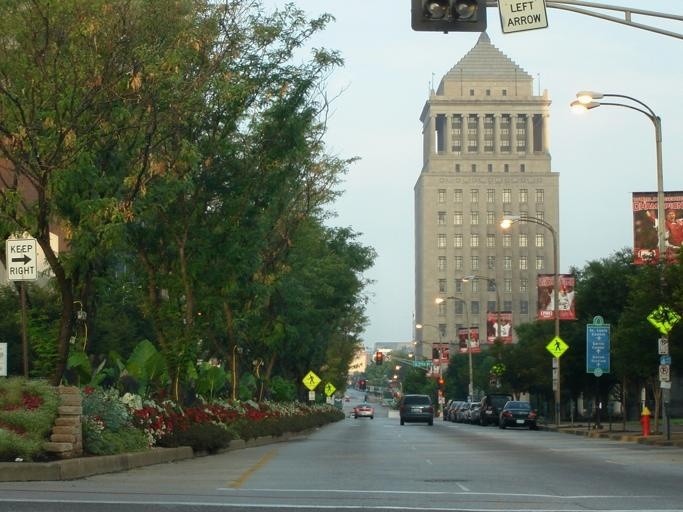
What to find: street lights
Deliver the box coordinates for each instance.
[434,294,475,414]
[568,90,675,438]
[460,275,502,421]
[387,322,443,414]
[498,212,559,427]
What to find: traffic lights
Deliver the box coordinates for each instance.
[359,380,365,390]
[432,347,438,359]
[412,0,486,30]
[375,352,382,365]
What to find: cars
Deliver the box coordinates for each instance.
[443,394,536,429]
[354,403,374,419]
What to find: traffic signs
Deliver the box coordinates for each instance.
[497,0,548,34]
[7,243,35,278]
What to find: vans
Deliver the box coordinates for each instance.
[399,394,433,426]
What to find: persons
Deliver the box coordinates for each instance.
[326,384,333,394]
[437,347,449,359]
[491,319,512,337]
[542,286,575,310]
[307,373,316,385]
[464,332,480,348]
[646,207,683,262]
[554,339,561,352]
[660,307,671,326]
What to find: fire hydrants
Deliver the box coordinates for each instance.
[639,406,651,436]
